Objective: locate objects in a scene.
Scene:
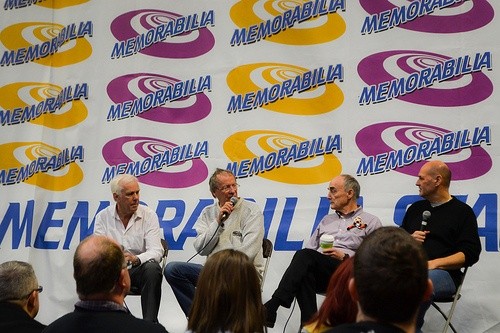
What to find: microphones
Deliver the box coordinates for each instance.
[121,245,129,266]
[222,197,237,219]
[420,211,431,231]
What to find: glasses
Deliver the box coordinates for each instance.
[26,284,44,300]
[121,260,133,272]
[214,183,240,193]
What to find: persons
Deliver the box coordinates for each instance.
[304,257,354,333]
[93,173,163,323]
[396,160,482,333]
[315,228,433,333]
[263,175,381,333]
[184,249,266,333]
[47,236,167,333]
[163,170,264,325]
[0,261,49,333]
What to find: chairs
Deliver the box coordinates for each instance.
[431,267,468,333]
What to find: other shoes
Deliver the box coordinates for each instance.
[261,305,277,328]
[153,320,168,332]
[298,325,303,332]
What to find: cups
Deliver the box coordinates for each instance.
[320,235,334,250]
